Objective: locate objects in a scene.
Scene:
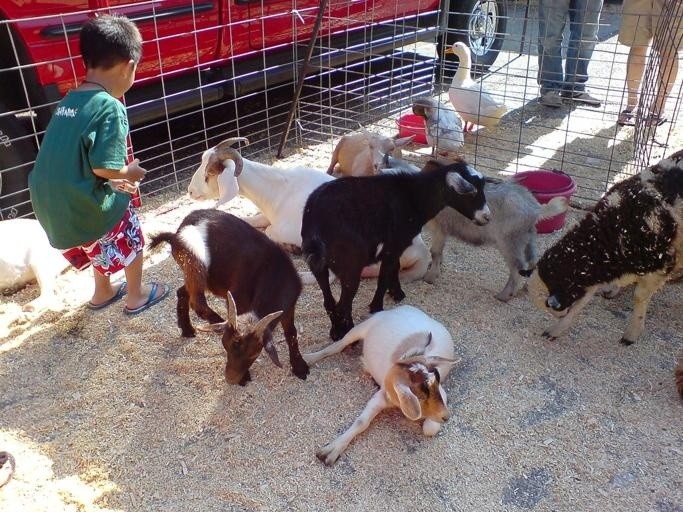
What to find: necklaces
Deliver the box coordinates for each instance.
[80,79,108,93]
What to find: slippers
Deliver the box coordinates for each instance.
[642,113,668,126]
[87,280,128,312]
[123,281,169,316]
[618,109,638,126]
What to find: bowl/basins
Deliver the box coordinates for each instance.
[395,113,429,145]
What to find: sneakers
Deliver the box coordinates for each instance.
[561,89,602,104]
[541,91,563,108]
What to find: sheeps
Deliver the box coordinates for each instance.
[0,218,69,322]
[301,304,461,466]
[187,137,433,284]
[518,150,683,347]
[301,162,492,342]
[374,150,553,303]
[327,121,421,177]
[146,208,310,386]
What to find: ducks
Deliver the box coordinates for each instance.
[444,42,507,135]
[412,97,464,157]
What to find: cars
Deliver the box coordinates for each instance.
[1,1,505,217]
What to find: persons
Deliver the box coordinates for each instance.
[615,0,683,127]
[26,12,169,317]
[537,0,604,107]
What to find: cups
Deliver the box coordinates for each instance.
[124,176,146,194]
